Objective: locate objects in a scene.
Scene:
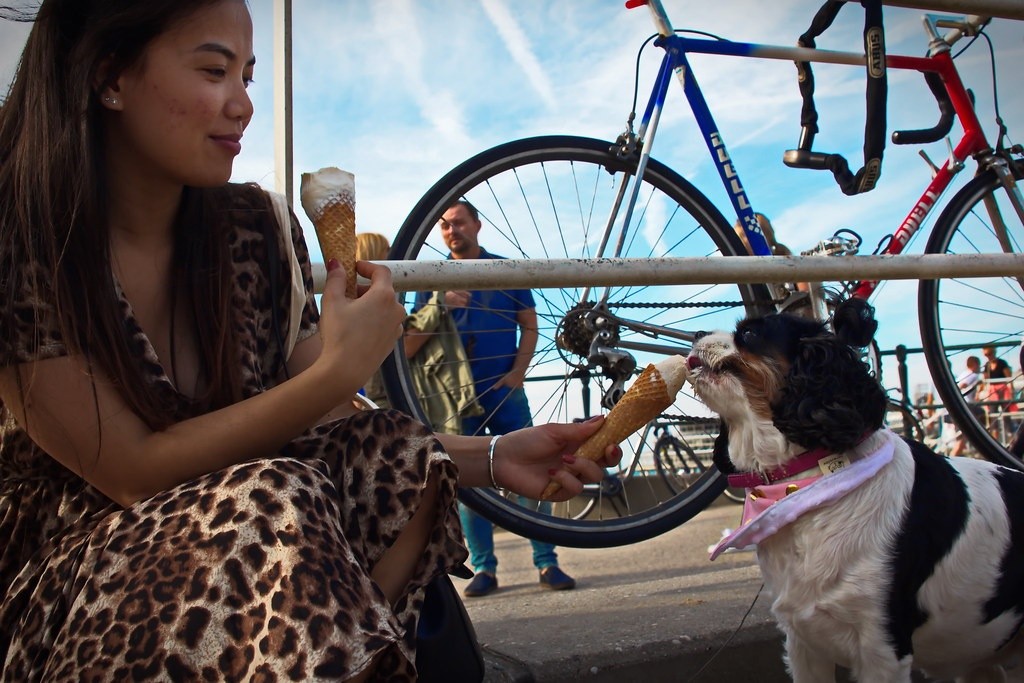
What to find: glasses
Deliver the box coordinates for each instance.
[985,350,995,357]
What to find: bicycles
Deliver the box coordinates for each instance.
[720,388,925,505]
[493,417,706,521]
[367,1,1024,549]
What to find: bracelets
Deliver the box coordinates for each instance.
[488,435,505,491]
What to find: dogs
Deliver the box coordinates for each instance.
[683,297,1024,683]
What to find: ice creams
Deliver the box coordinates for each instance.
[299,168,358,303]
[541,353,688,502]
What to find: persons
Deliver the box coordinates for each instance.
[1,0,622,682]
[914,342,1023,461]
[412,200,575,595]
[736,213,813,319]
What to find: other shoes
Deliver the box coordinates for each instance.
[464,572,498,597]
[540,566,575,591]
